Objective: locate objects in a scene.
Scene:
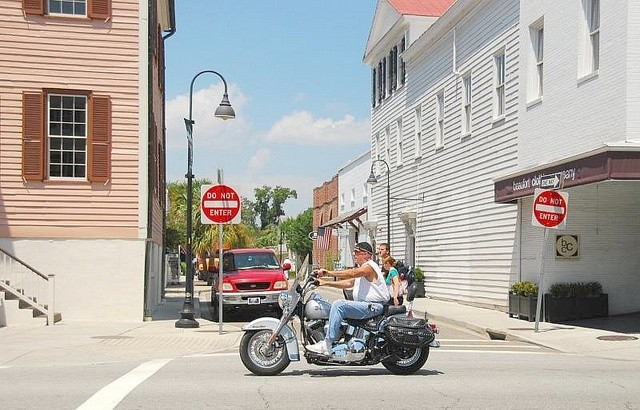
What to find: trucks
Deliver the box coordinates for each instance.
[198,244,230,285]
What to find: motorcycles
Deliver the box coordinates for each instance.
[239,252,441,376]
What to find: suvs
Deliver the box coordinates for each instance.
[208,247,291,321]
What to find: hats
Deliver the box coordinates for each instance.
[354,242,373,255]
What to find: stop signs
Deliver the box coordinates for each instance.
[532,187,569,228]
[200,184,241,225]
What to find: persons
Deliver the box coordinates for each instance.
[379,243,391,279]
[305,241,391,358]
[382,256,405,306]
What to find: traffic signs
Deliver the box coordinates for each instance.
[540,175,561,188]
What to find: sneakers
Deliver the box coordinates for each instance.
[305,341,333,356]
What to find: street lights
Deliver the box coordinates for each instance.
[367,159,390,261]
[175,70,236,328]
